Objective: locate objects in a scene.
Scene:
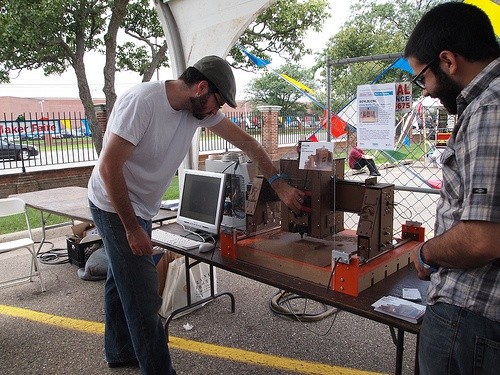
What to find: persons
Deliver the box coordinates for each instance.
[87,55,312,375]
[349,141,381,176]
[402,2,500,375]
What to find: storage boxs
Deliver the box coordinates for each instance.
[65,222,104,269]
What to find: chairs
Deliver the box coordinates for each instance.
[0,197,46,293]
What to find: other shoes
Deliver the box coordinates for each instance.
[370,172,377,176]
[376,172,381,176]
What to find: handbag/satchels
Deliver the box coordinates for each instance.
[151,246,218,318]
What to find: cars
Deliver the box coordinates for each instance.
[0,138,40,162]
[62,127,92,139]
[230,115,321,131]
[417,117,434,128]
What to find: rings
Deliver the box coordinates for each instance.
[298,198,301,201]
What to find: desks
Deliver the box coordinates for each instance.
[8,185,177,272]
[151,222,430,375]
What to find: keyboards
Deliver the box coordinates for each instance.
[150,228,202,251]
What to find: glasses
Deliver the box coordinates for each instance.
[209,85,223,110]
[412,56,439,90]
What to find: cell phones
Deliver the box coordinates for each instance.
[402,288,423,301]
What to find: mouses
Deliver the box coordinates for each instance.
[198,242,215,253]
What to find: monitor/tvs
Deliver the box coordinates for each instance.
[176,168,227,234]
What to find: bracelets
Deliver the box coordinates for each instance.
[267,173,283,186]
[418,240,438,273]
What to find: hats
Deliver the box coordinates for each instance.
[193,55,237,108]
